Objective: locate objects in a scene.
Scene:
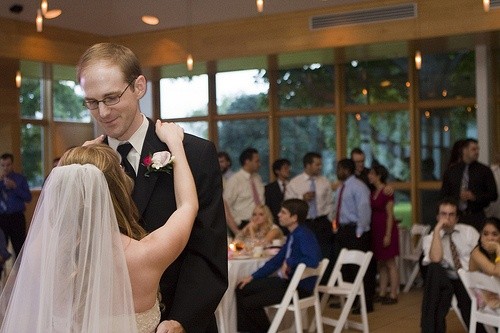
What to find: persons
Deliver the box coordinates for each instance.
[222,147,265,239]
[420,199,500,333]
[234,199,327,333]
[332,158,377,315]
[283,152,334,253]
[264,158,292,236]
[235,204,283,245]
[329,148,394,197]
[217,151,233,183]
[367,165,400,306]
[77,42,229,333]
[0,153,33,279]
[443,137,498,230]
[0,119,198,333]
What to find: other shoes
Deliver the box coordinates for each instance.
[374,295,400,306]
[352,305,375,315]
[329,300,356,309]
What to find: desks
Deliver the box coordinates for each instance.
[213,256,270,333]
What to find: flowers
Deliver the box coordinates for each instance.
[142,149,175,178]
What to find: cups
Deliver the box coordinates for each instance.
[235,236,244,251]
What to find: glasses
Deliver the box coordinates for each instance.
[82,78,137,109]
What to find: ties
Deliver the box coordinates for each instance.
[277,235,294,280]
[282,183,287,197]
[309,177,317,224]
[116,142,137,181]
[336,184,346,229]
[458,165,469,210]
[446,232,462,274]
[250,176,262,207]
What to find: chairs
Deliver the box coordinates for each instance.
[396,223,500,333]
[267,247,374,333]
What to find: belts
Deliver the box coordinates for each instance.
[305,215,328,224]
[339,223,357,230]
